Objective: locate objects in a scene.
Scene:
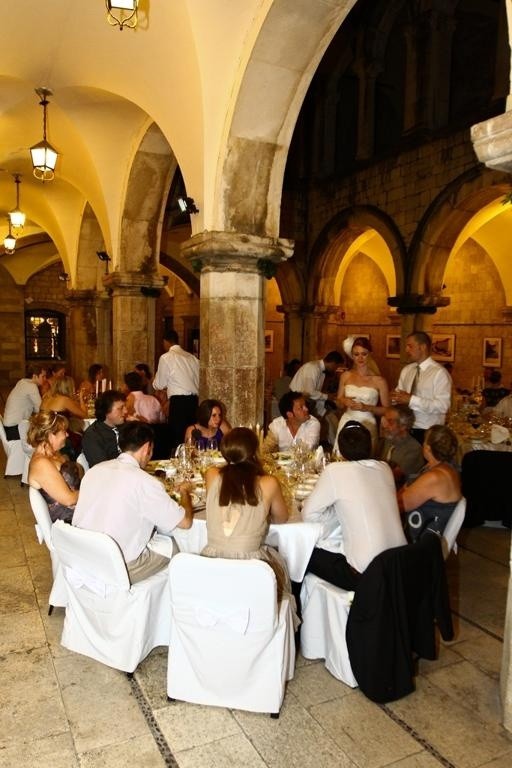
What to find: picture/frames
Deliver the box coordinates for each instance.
[264,329,279,351]
[350,332,370,343]
[386,334,401,359]
[482,337,503,368]
[431,332,457,362]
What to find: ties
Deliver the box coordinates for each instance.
[409,365,420,395]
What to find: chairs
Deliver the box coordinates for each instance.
[54,518,171,681]
[461,441,511,531]
[299,539,438,690]
[1,410,468,485]
[167,551,295,720]
[413,495,467,557]
[29,487,71,618]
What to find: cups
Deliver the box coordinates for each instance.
[391,388,399,405]
[449,383,511,446]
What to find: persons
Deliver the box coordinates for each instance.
[67,420,194,585]
[0,326,201,468]
[478,369,511,421]
[199,425,293,608]
[269,330,452,486]
[23,407,85,525]
[396,422,466,551]
[300,417,409,595]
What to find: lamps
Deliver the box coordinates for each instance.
[2,217,19,258]
[8,172,26,233]
[105,0,141,35]
[29,85,60,181]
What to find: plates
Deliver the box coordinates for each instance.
[149,437,331,512]
